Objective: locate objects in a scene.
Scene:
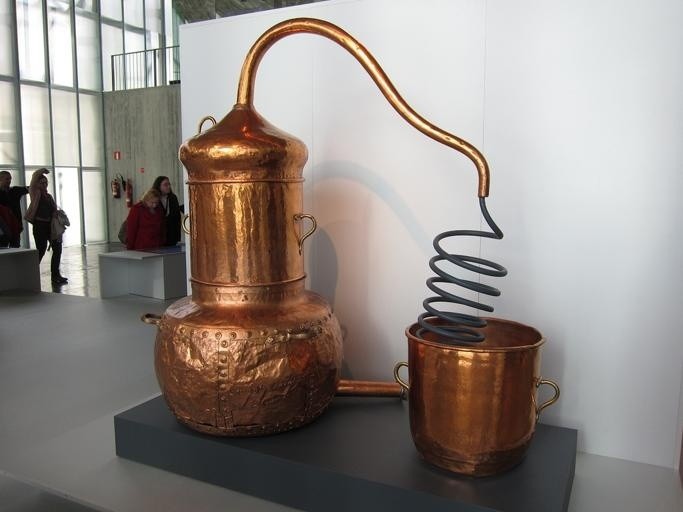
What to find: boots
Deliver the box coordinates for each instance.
[51,269,68,282]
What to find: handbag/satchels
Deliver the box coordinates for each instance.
[118,221,126,245]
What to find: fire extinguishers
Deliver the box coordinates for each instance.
[110,173,132,208]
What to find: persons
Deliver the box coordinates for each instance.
[152,176,182,246]
[127,189,165,249]
[0,171,30,248]
[24,168,68,283]
